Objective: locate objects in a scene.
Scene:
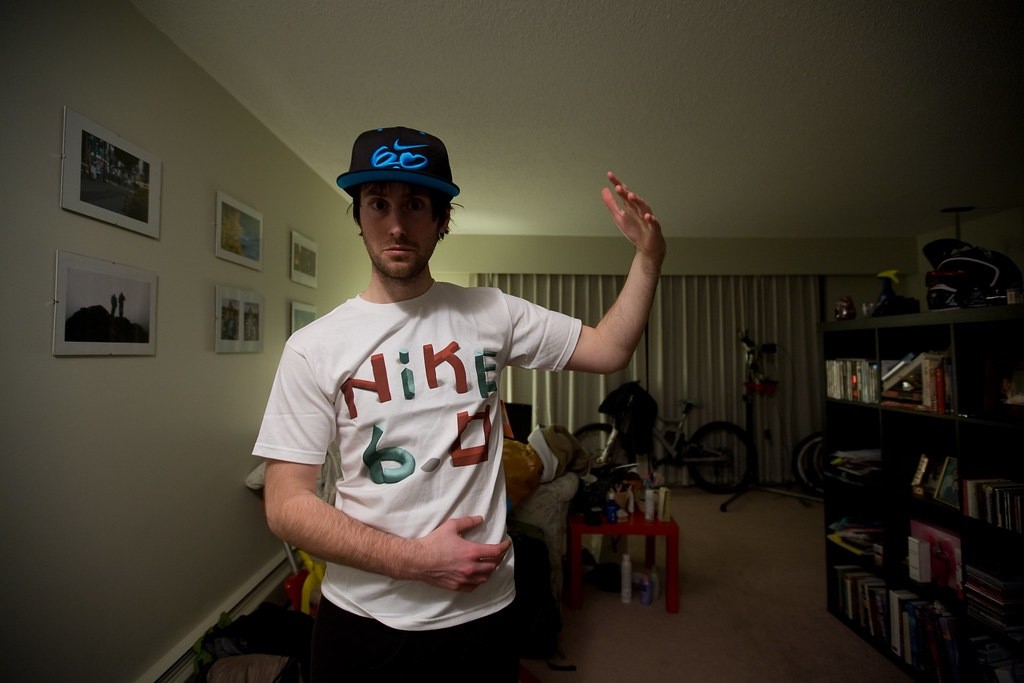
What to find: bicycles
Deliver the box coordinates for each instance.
[573,380,760,495]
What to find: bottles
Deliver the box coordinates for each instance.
[639,575,650,606]
[621,555,631,602]
[834,296,856,320]
[649,565,660,602]
[644,489,655,521]
[607,493,616,523]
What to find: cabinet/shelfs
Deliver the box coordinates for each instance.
[815,304,1024,683]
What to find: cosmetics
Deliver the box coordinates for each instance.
[607,493,616,523]
[616,509,628,523]
[644,489,657,523]
[626,489,635,516]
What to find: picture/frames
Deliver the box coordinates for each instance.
[60,123,161,241]
[289,230,321,291]
[215,190,264,270]
[934,456,959,509]
[290,300,318,336]
[215,284,263,354]
[50,250,158,355]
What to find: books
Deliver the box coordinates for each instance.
[828,518,886,564]
[880,350,956,414]
[834,565,1024,683]
[829,449,882,487]
[823,358,897,406]
[958,475,1024,535]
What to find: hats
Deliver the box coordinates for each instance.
[336,126,460,198]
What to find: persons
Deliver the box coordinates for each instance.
[250,127,666,683]
[939,466,961,505]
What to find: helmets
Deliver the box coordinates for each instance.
[927,247,1024,311]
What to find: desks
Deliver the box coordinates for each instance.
[565,511,680,615]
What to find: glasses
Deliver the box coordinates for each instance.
[924,270,980,292]
